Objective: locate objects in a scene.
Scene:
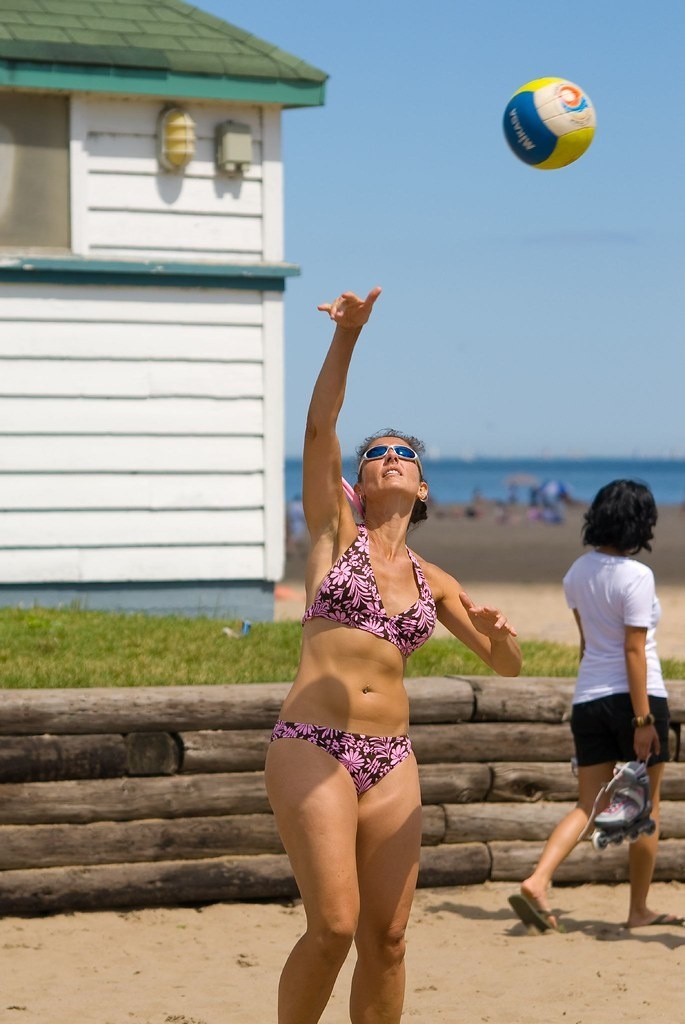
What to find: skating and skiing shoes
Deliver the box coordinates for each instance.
[578,752,657,851]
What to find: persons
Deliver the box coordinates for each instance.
[505,474,685,935]
[261,283,527,1024]
[462,471,575,524]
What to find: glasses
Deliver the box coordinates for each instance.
[358,445,423,482]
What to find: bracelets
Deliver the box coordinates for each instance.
[630,713,656,728]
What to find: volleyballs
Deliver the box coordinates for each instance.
[502,75,598,171]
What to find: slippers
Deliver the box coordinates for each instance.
[507,894,566,934]
[624,914,685,928]
